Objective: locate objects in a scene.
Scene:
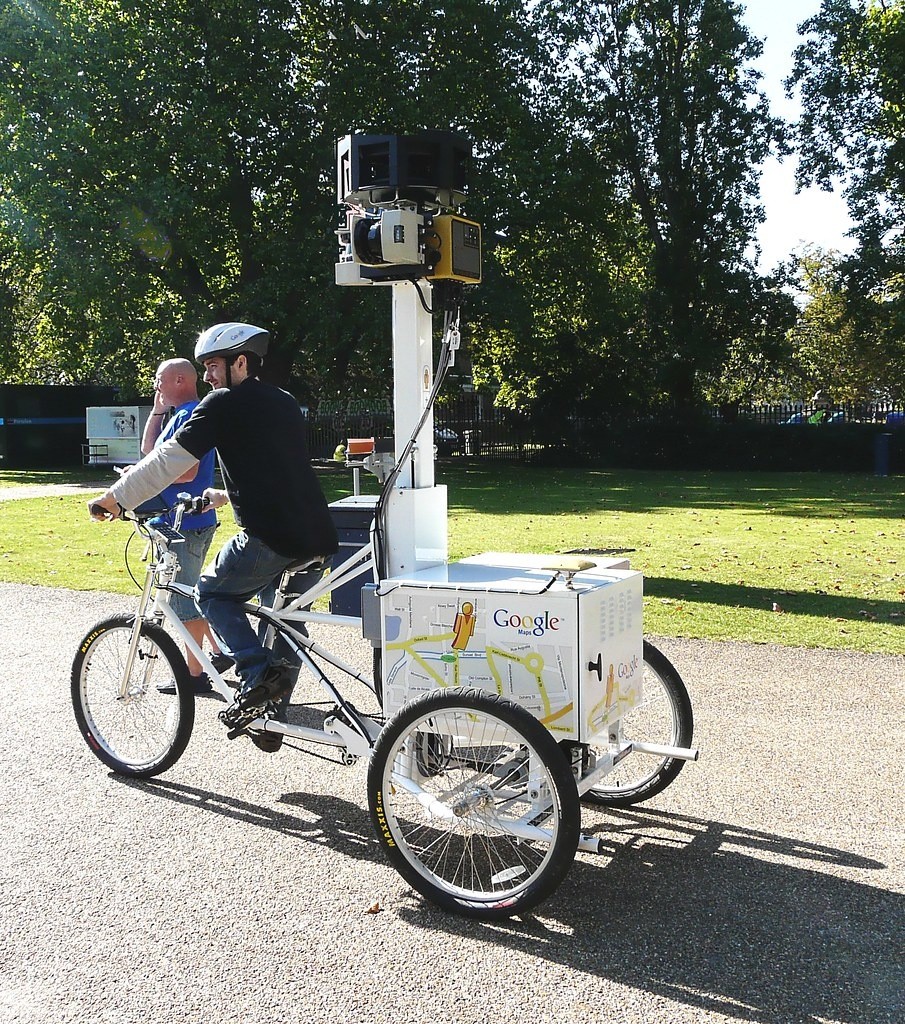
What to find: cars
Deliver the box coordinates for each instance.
[779,408,905,426]
[433,423,460,459]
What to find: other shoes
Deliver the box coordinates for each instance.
[158,672,213,694]
[209,653,235,674]
[219,671,291,721]
[220,711,283,752]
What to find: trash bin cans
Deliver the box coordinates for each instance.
[327,494,385,617]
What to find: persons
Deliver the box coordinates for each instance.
[87,322,338,753]
[119,358,235,695]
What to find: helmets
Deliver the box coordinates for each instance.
[194,323,269,363]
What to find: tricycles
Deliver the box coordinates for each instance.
[70,488,700,920]
